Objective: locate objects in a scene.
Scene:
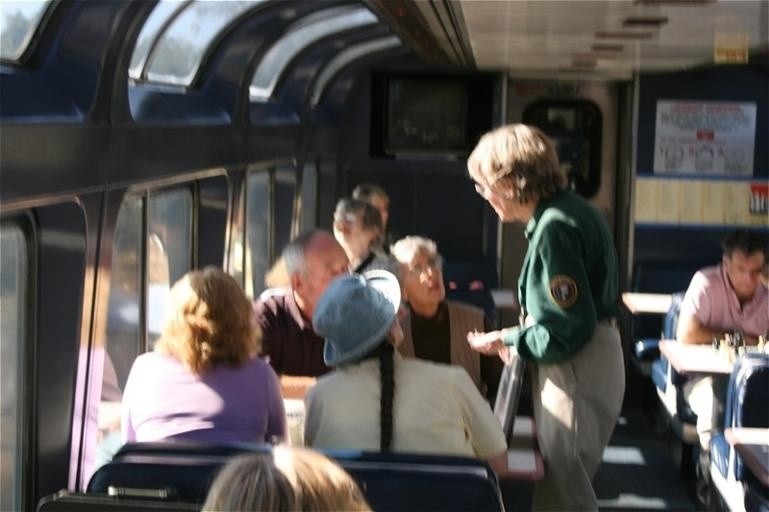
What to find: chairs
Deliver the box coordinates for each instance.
[736,361,769,511]
[668,360,700,445]
[630,262,696,360]
[710,353,769,498]
[652,291,683,403]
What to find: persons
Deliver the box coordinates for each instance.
[352,180,409,252]
[247,227,407,402]
[200,442,374,511]
[335,196,393,275]
[123,262,291,449]
[676,226,769,512]
[303,271,509,481]
[464,122,626,511]
[384,235,506,408]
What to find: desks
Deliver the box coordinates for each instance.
[621,292,672,314]
[659,340,769,374]
[724,427,769,445]
[39,289,543,511]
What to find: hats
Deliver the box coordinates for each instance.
[312,269,402,366]
